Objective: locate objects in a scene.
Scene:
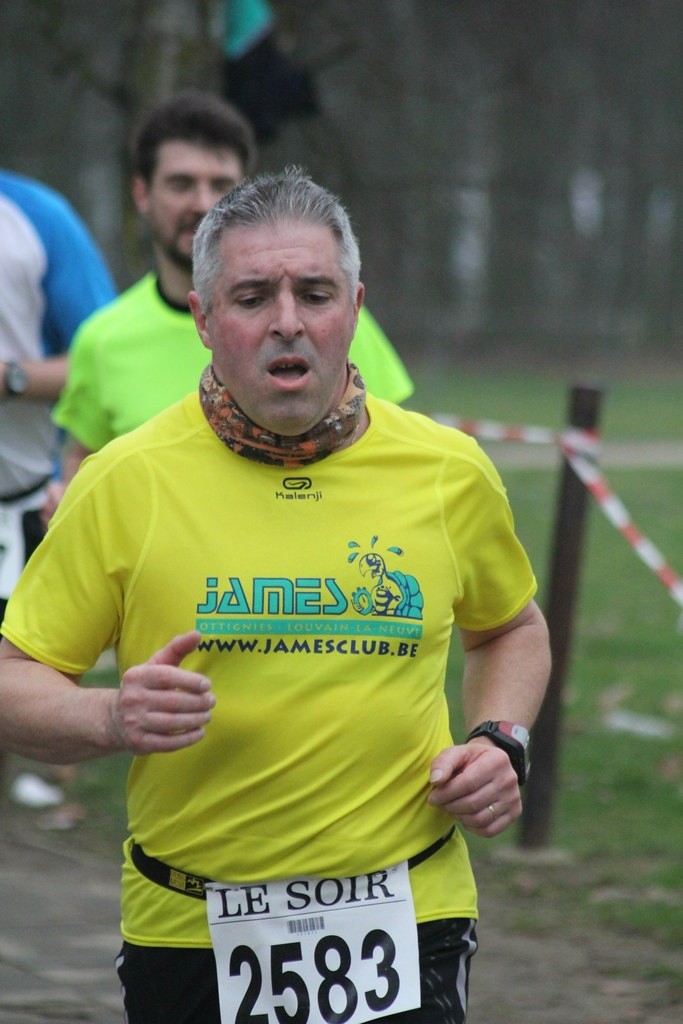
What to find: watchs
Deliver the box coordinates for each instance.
[3,360,28,400]
[465,720,534,785]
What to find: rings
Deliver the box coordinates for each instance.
[487,805,497,820]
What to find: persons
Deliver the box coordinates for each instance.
[0,163,554,1024]
[0,172,119,800]
[43,96,419,528]
